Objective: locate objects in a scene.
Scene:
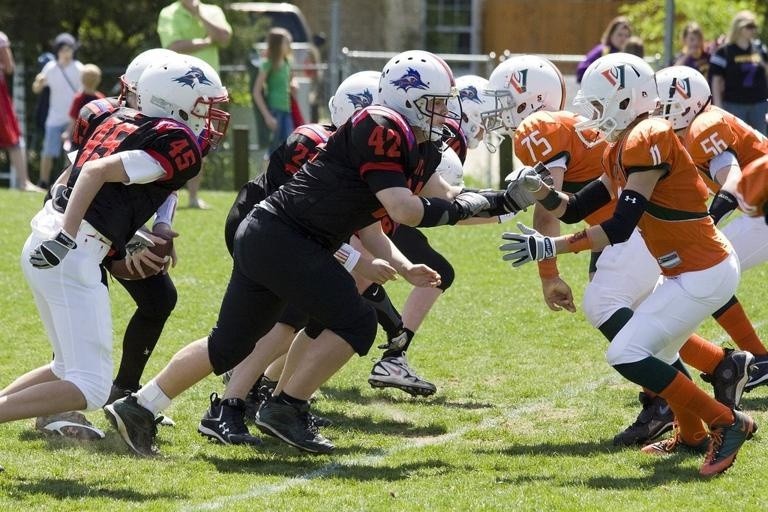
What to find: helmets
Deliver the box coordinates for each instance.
[446,75,504,150]
[118,48,178,109]
[140,55,231,158]
[481,53,565,154]
[656,66,713,139]
[573,52,659,148]
[327,71,382,128]
[380,50,463,153]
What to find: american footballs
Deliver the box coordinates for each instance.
[111,233,172,280]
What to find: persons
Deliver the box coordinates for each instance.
[103,52,545,460]
[0,56,230,423]
[221,138,464,430]
[0,31,45,193]
[33,49,178,441]
[648,64,768,393]
[63,64,108,131]
[156,1,233,209]
[674,26,711,85]
[32,33,85,191]
[713,12,768,139]
[196,69,441,447]
[574,18,631,83]
[253,26,293,174]
[365,74,508,398]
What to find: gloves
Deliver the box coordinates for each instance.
[455,192,490,220]
[28,227,77,270]
[506,166,542,192]
[378,325,409,356]
[503,162,552,215]
[499,222,556,268]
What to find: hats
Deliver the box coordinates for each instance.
[735,11,755,30]
[54,32,76,55]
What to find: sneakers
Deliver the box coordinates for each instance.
[102,390,168,460]
[243,386,263,422]
[260,376,277,394]
[258,396,333,428]
[744,353,768,392]
[102,382,177,427]
[640,433,713,455]
[222,369,234,385]
[614,393,680,446]
[368,351,437,397]
[699,398,757,476]
[197,392,262,445]
[701,346,754,410]
[255,400,335,453]
[35,411,105,440]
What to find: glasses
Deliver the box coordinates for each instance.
[747,24,757,29]
